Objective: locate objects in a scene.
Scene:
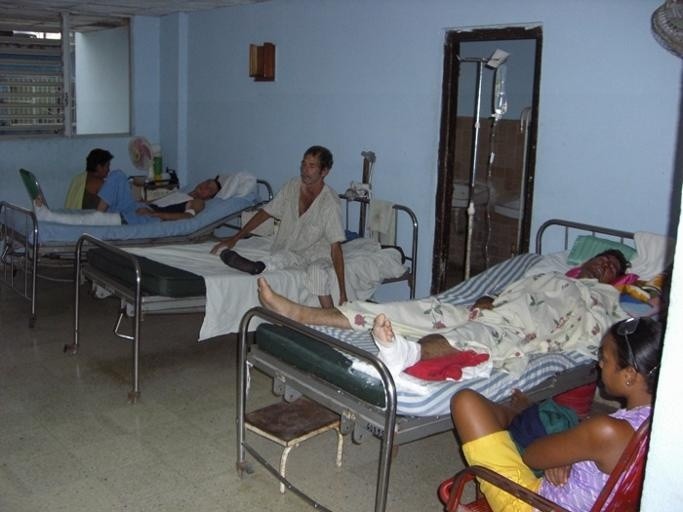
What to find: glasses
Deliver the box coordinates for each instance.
[617,316,642,370]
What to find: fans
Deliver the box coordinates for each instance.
[126,135,163,184]
[649,1,682,59]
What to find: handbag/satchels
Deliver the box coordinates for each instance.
[508,398,578,479]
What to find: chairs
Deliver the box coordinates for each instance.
[17,169,49,212]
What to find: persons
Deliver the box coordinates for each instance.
[31,178,224,227]
[63,146,112,210]
[447,315,663,512]
[206,142,347,308]
[247,244,634,377]
